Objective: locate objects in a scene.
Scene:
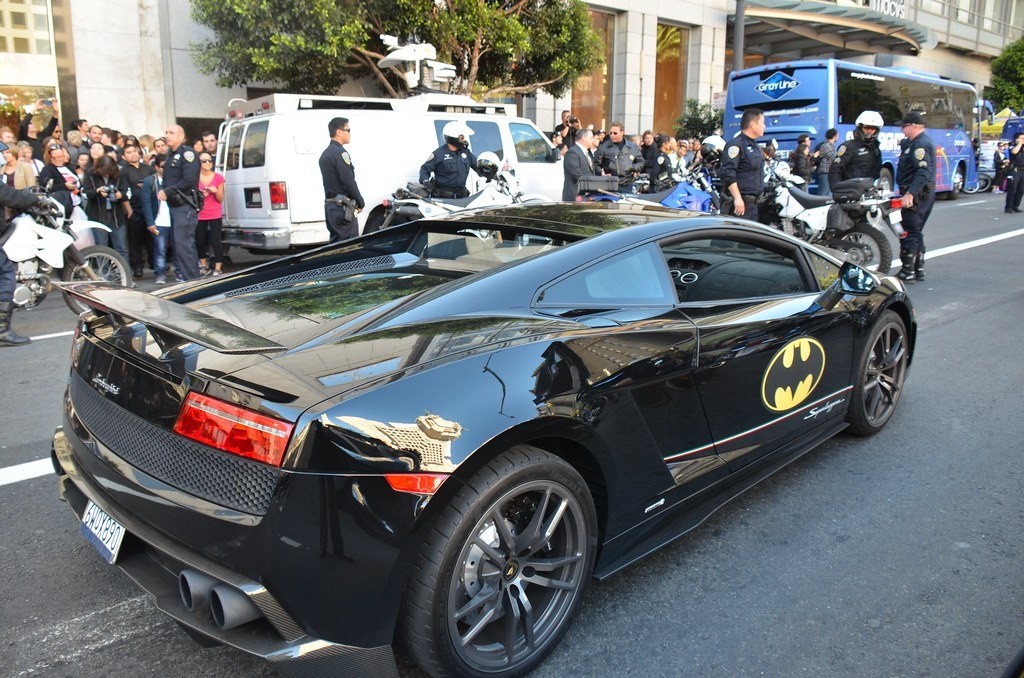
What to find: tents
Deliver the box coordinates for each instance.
[974,108,1020,135]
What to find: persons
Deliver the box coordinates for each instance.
[0,96,225,284]
[319,117,365,243]
[763,129,839,198]
[992,132,1024,213]
[719,108,766,223]
[828,111,884,242]
[550,110,705,201]
[896,112,936,283]
[0,140,59,346]
[418,121,490,199]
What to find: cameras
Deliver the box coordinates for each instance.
[570,119,577,123]
[104,184,118,201]
[43,100,52,106]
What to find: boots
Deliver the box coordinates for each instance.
[915,251,926,280]
[0,301,31,347]
[896,253,917,284]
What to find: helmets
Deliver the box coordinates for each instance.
[856,110,884,142]
[443,121,475,150]
[701,135,726,155]
[477,151,500,183]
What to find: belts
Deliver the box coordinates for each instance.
[326,197,335,203]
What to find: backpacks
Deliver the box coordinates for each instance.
[811,141,829,166]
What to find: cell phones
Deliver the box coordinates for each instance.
[72,180,77,185]
[204,187,210,192]
[154,229,159,236]
[138,180,143,183]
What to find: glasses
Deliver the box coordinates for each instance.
[861,124,876,130]
[342,129,350,132]
[49,144,61,152]
[201,159,212,163]
[54,130,62,133]
[902,124,912,128]
[610,132,617,135]
[158,165,164,168]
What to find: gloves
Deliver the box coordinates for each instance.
[423,181,433,193]
[34,198,59,211]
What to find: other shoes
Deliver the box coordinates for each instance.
[199,266,211,278]
[155,273,167,284]
[992,189,1004,194]
[134,264,144,277]
[1004,207,1022,213]
[213,269,222,276]
[175,273,188,282]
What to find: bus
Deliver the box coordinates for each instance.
[722,58,994,200]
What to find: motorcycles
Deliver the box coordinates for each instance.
[380,158,535,229]
[765,161,908,275]
[574,148,721,216]
[0,178,135,316]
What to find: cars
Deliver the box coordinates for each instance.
[980,110,1024,191]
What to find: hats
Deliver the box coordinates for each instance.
[894,111,925,125]
[550,130,561,138]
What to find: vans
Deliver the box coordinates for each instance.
[213,90,566,253]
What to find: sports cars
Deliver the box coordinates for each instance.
[49,200,916,678]
